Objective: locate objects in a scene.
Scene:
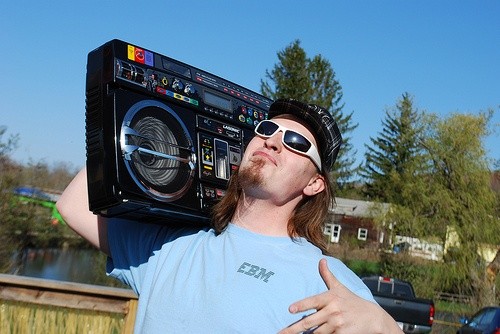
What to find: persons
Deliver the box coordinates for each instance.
[55,99,404,334]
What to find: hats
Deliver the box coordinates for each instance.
[267,98,342,174]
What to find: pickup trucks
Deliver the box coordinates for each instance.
[359,275,436,334]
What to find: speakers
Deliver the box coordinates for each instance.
[85,38,274,229]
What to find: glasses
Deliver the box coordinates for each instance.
[254,120,322,173]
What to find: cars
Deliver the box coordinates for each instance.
[455,306,500,334]
